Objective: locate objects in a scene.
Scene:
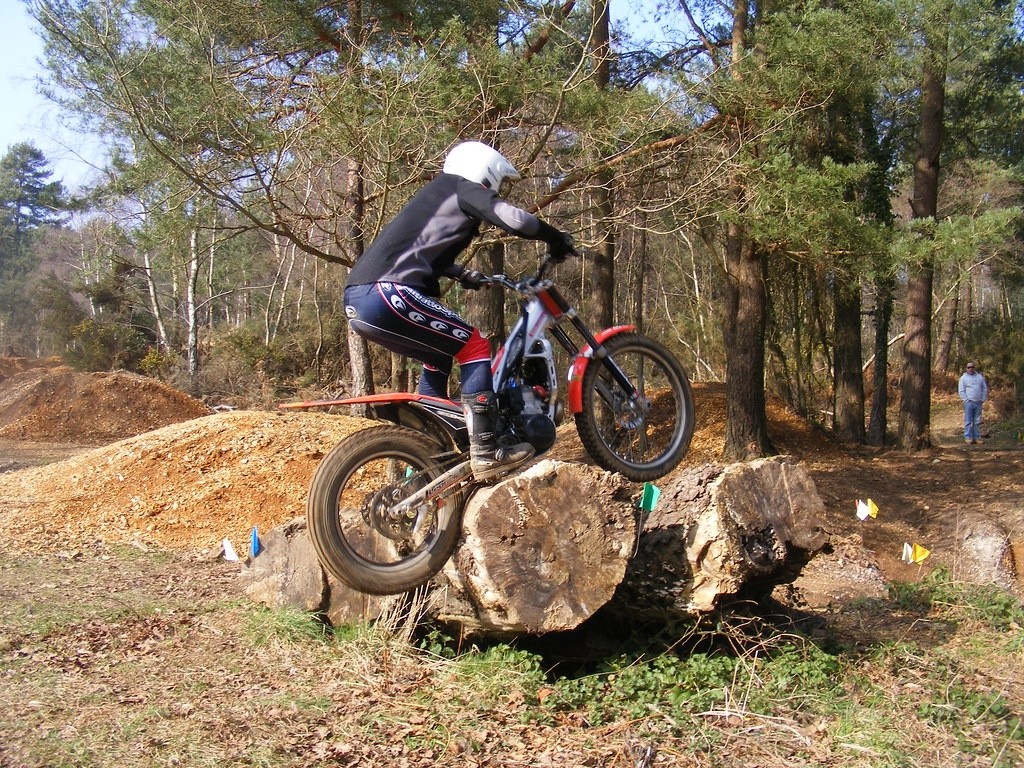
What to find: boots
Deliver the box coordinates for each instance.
[461,390,536,481]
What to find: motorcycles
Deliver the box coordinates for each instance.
[275,231,698,598]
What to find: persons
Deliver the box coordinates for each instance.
[958,361,987,444]
[344,141,574,480]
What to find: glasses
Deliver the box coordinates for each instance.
[967,366,975,369]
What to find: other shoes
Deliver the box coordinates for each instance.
[965,439,973,444]
[975,440,983,445]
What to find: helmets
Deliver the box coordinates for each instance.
[443,140,522,195]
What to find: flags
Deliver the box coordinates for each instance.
[639,483,661,510]
[1013,430,1022,439]
[252,527,260,558]
[867,498,879,518]
[910,543,931,565]
[223,538,240,561]
[856,500,871,521]
[902,542,915,565]
